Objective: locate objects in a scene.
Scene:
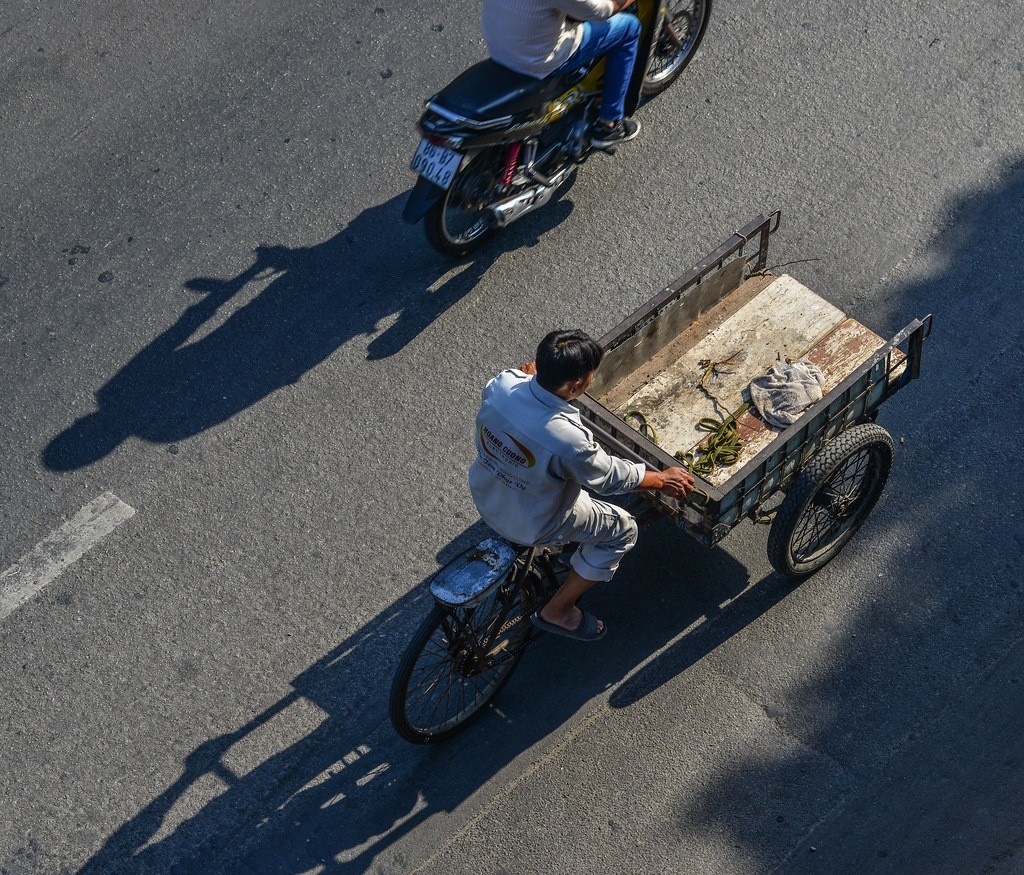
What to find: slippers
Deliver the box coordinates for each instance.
[530,609,608,641]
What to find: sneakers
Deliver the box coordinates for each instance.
[591,119,641,147]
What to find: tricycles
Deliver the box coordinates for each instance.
[388,208,935,747]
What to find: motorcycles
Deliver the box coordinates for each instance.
[401,0,712,258]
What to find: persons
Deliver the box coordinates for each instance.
[480,0,641,150]
[469,330,695,640]
